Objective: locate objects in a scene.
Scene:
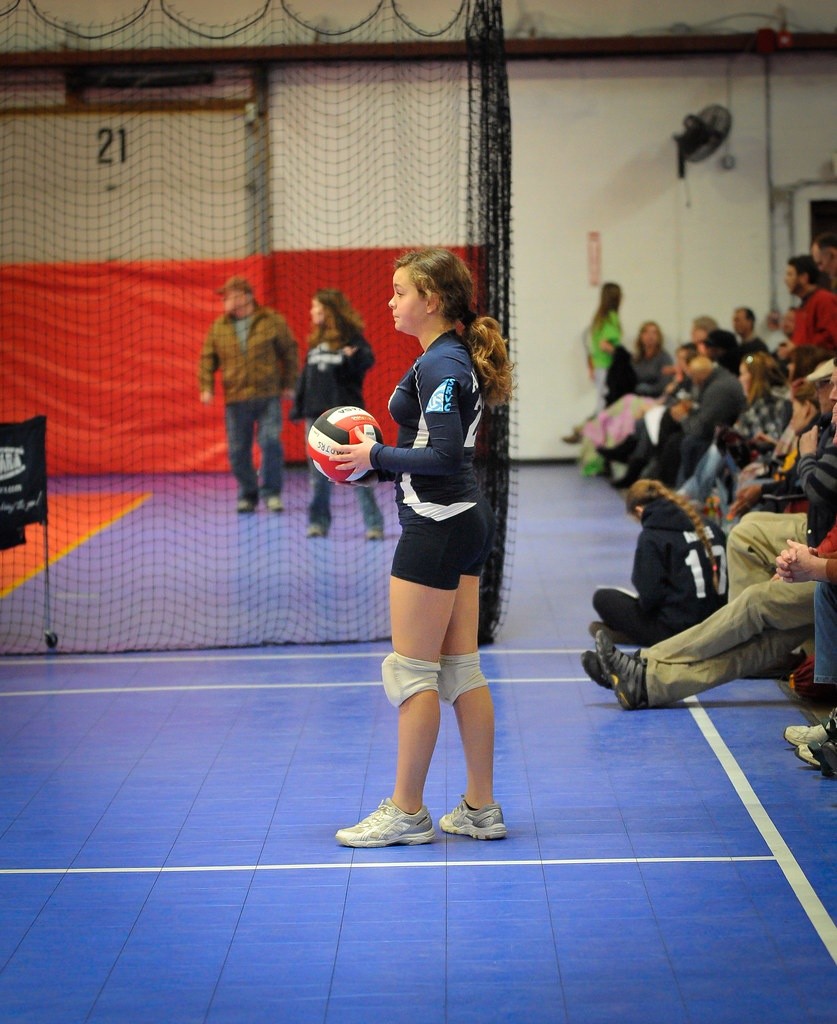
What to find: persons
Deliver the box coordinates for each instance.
[198,275,299,512]
[289,290,384,539]
[562,231,837,778]
[329,249,512,848]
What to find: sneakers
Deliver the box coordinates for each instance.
[596,630,643,710]
[335,796,435,848]
[784,725,837,778]
[582,649,636,689]
[436,796,504,839]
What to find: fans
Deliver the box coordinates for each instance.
[672,105,731,178]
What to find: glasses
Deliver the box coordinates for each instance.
[812,380,831,393]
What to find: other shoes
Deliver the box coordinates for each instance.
[268,495,284,513]
[589,624,629,646]
[563,430,581,442]
[306,523,322,537]
[236,499,255,511]
[612,456,641,489]
[596,445,628,464]
[365,528,383,541]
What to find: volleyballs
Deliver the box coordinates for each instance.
[304,404,385,485]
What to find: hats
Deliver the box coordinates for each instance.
[214,276,250,295]
[805,358,836,381]
